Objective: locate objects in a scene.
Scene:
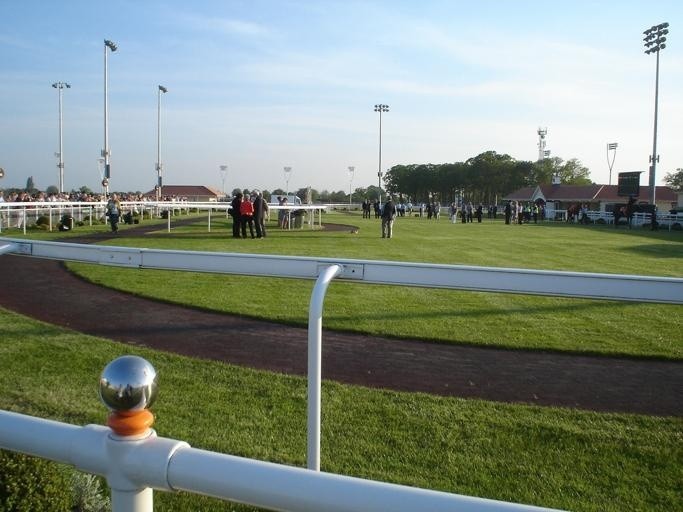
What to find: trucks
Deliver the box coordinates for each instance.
[270,195,302,204]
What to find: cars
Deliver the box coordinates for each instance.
[587,212,683,231]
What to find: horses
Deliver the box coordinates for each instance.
[566,203,582,224]
[612,195,637,226]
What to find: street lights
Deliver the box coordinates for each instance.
[374,103,390,205]
[101,38,119,197]
[282,167,292,194]
[346,166,356,203]
[155,84,168,197]
[218,164,228,193]
[51,80,72,193]
[642,21,669,204]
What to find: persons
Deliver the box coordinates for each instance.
[277,195,291,230]
[380,195,397,238]
[581,203,589,225]
[227,189,270,240]
[0,190,188,228]
[105,193,122,233]
[361,197,547,224]
